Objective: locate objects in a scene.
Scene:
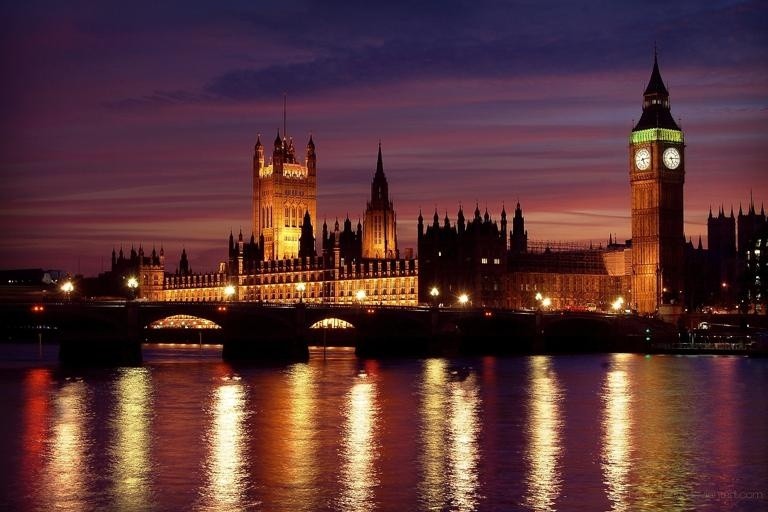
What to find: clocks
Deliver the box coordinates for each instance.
[633,147,653,172]
[662,147,681,169]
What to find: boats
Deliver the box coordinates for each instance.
[657,321,768,355]
